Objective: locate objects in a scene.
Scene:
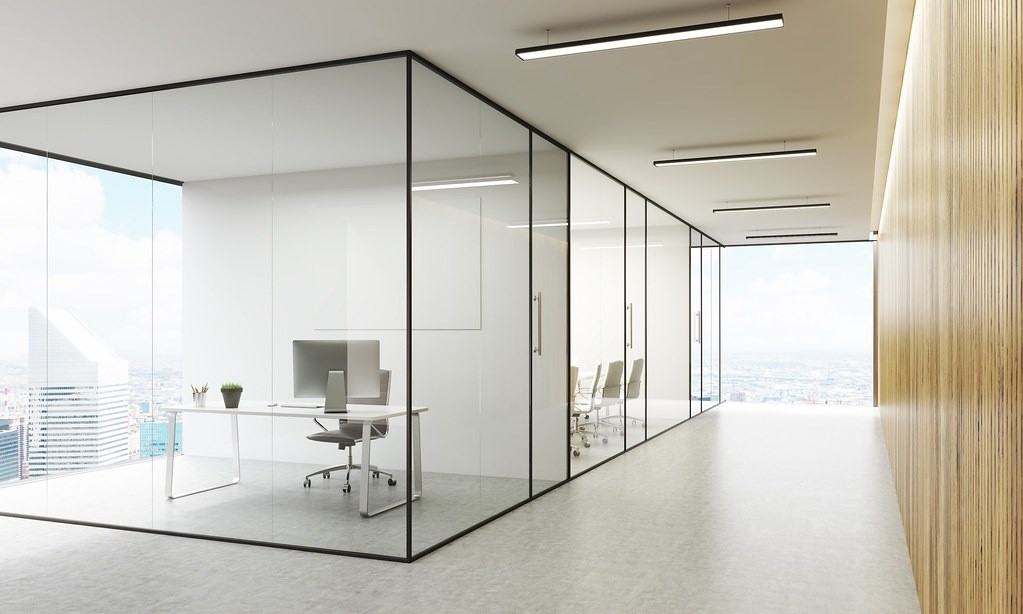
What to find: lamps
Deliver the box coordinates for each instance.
[411,173,521,191]
[713,203,831,214]
[515,13,785,61]
[654,149,818,167]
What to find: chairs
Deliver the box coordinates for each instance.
[580,361,622,438]
[600,359,647,431]
[303,370,397,494]
[570,365,602,457]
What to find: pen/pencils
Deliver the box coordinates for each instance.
[191,382,210,394]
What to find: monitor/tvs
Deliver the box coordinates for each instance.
[293,339,380,414]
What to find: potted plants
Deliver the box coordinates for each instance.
[221,384,243,408]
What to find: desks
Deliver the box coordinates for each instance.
[160,402,428,518]
